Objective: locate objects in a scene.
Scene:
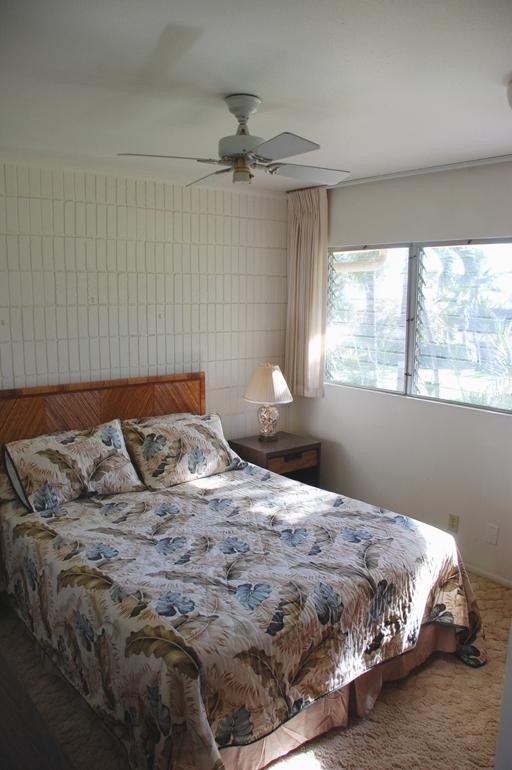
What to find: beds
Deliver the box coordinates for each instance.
[1,371,492,770]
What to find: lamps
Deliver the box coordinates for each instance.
[230,157,253,185]
[244,359,295,442]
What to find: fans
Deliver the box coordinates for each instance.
[117,92,354,188]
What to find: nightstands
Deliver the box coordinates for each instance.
[227,430,321,488]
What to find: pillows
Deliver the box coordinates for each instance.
[117,411,252,494]
[3,415,148,518]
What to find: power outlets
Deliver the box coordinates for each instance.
[446,511,461,534]
